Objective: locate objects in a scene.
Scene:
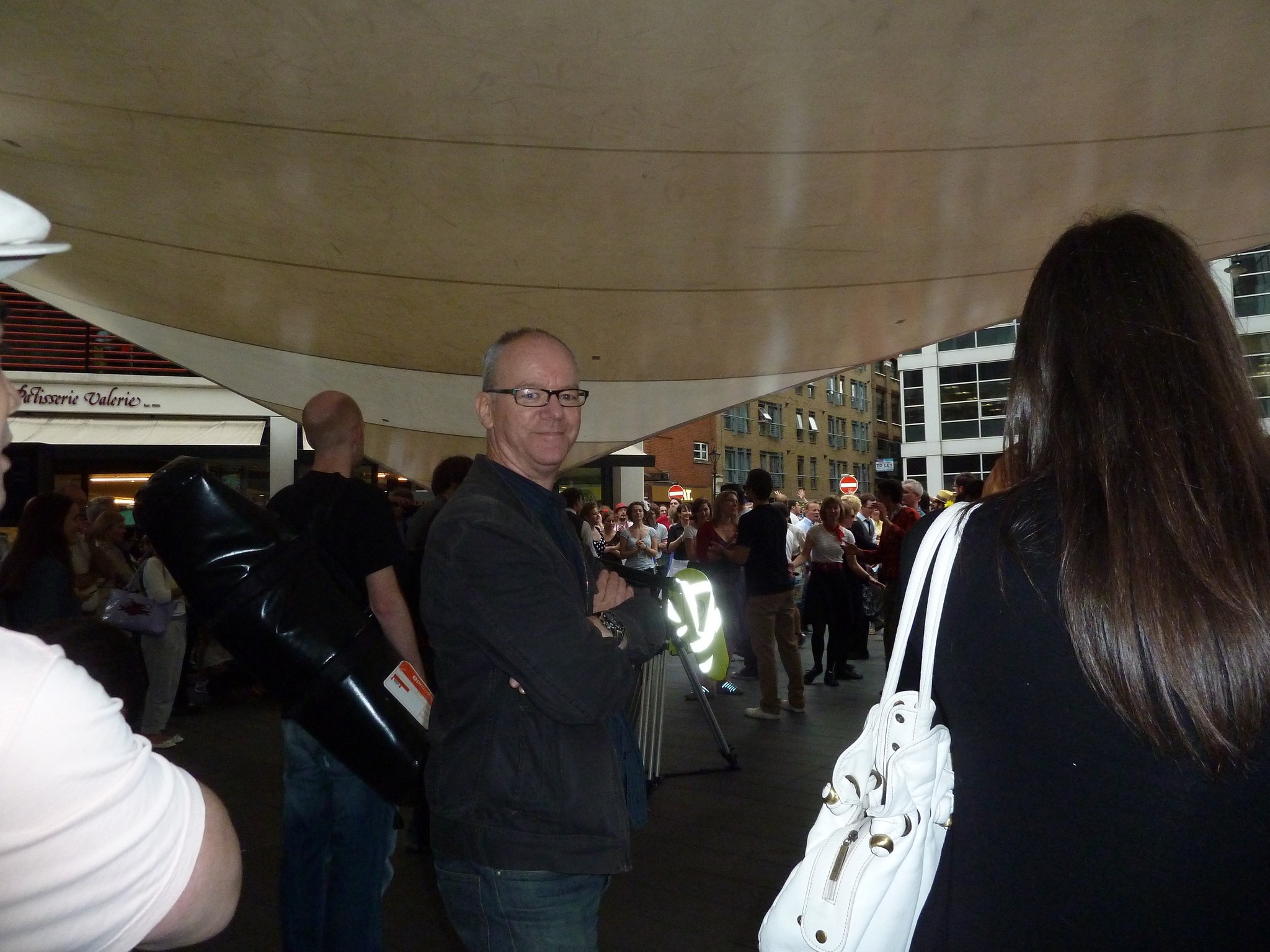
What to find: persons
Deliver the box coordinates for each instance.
[886,206,1268,952]
[1,449,475,757]
[0,290,247,948]
[250,391,429,942]
[556,444,1027,722]
[405,325,661,950]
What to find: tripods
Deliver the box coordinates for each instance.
[635,564,743,793]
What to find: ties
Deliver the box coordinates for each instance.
[864,519,872,535]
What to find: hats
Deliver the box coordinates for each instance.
[613,503,628,513]
[936,489,953,503]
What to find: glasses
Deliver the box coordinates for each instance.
[902,490,916,494]
[952,485,959,489]
[863,505,874,510]
[743,485,749,491]
[483,388,590,408]
[128,538,139,543]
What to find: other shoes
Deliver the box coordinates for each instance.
[404,833,431,853]
[848,650,870,660]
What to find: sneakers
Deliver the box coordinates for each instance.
[875,627,885,635]
[780,699,805,712]
[745,706,782,720]
[800,625,813,634]
[732,666,757,680]
[803,667,840,686]
[832,664,865,680]
[869,621,875,635]
[794,633,806,648]
[732,653,745,661]
[685,685,714,700]
[716,680,743,696]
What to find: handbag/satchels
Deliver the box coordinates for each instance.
[102,588,178,638]
[753,691,959,952]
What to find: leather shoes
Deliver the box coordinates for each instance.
[141,731,185,748]
